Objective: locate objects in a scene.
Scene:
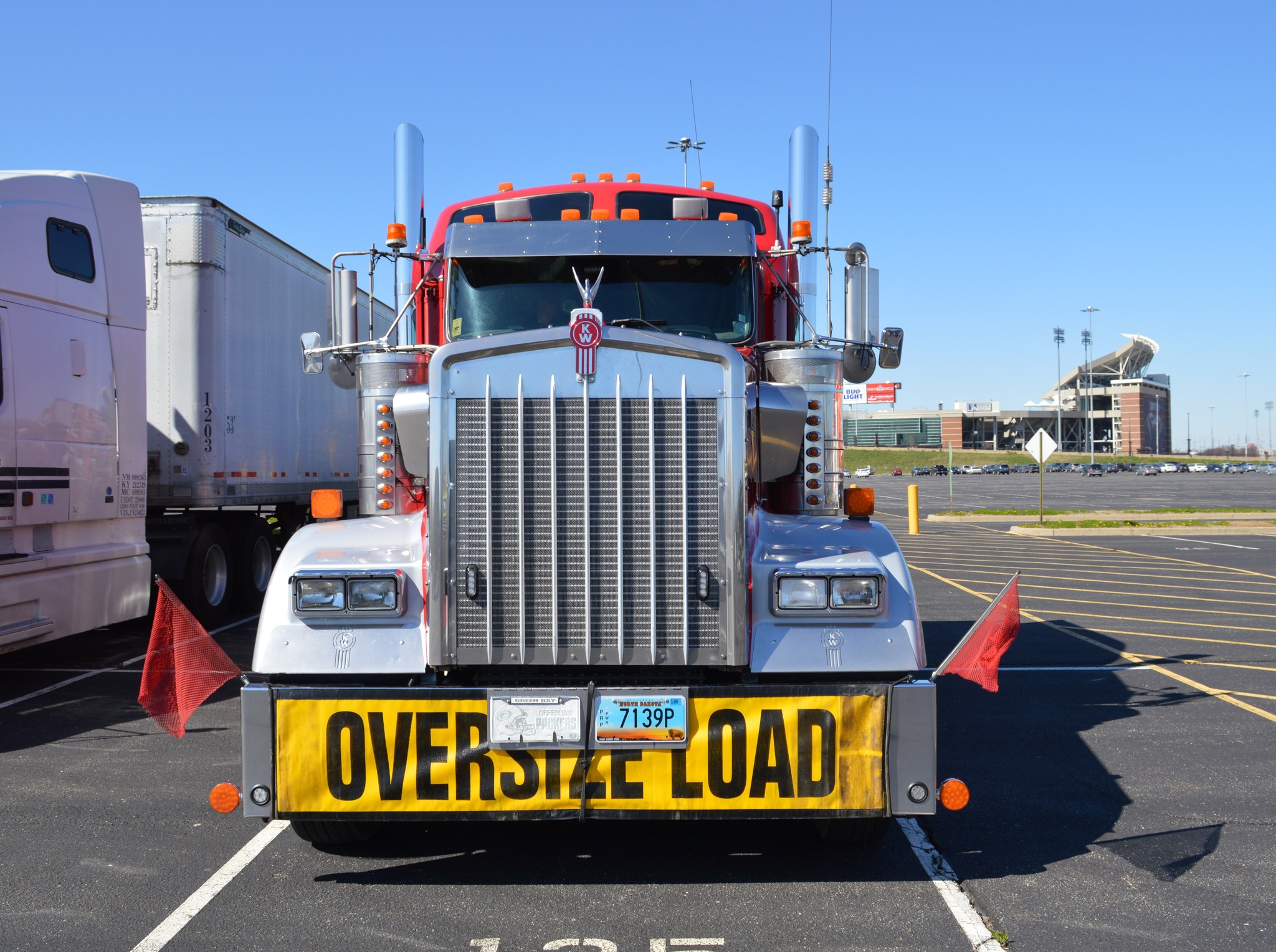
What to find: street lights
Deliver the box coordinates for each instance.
[1265,401,1276,454]
[1238,373,1250,458]
[1187,413,1191,454]
[1080,306,1101,465]
[1252,409,1260,455]
[1052,326,1067,453]
[1209,406,1215,454]
[1080,327,1095,454]
[1155,394,1161,455]
[973,410,978,450]
[665,136,707,190]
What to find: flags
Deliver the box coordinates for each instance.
[939,578,1020,692]
[138,579,242,739]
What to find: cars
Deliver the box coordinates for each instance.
[842,462,1276,477]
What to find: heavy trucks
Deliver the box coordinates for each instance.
[209,121,972,844]
[0,170,398,657]
